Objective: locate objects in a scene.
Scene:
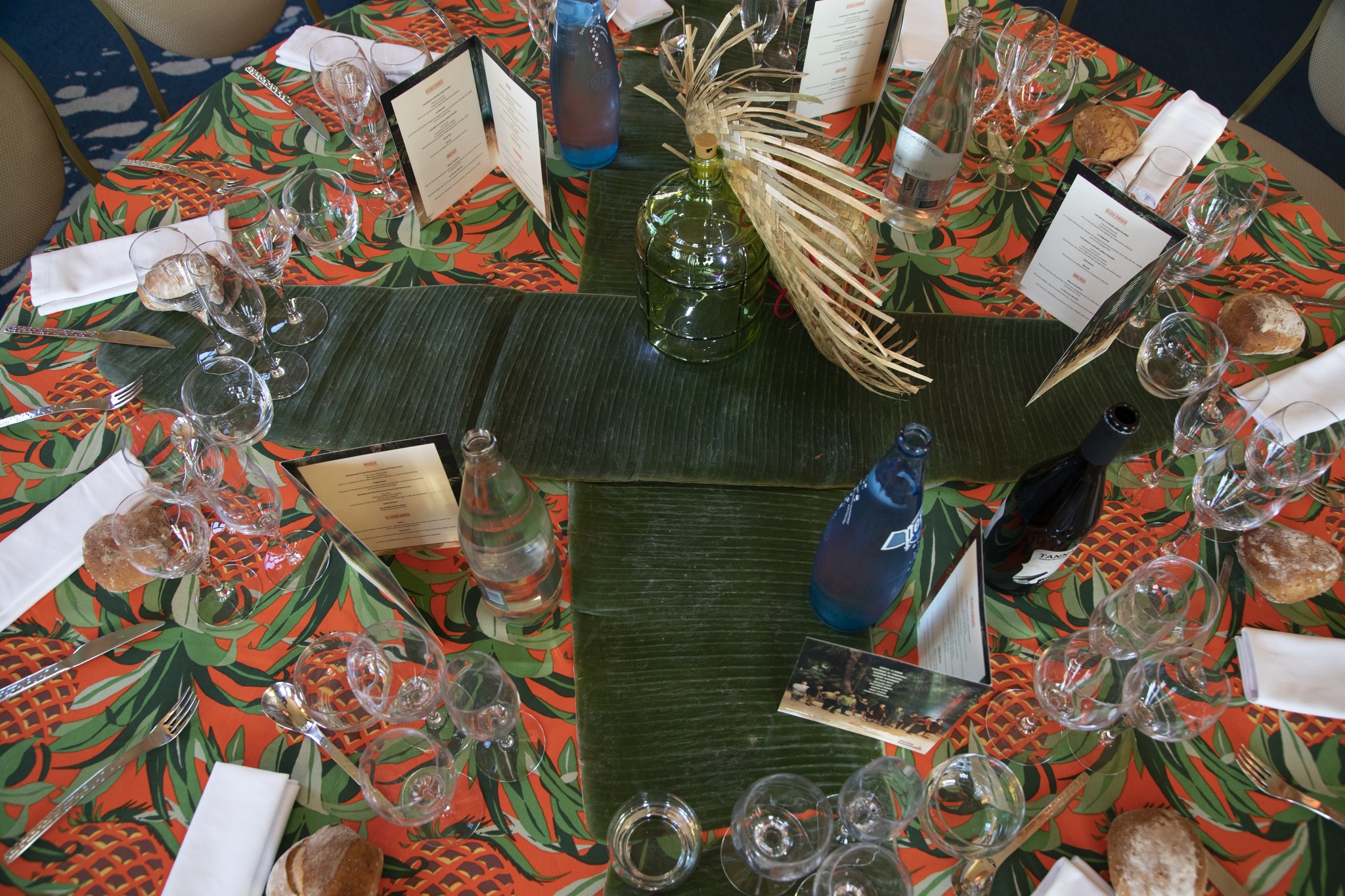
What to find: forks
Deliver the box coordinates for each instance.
[426,2,469,45]
[1235,740,1343,831]
[120,154,248,197]
[6,682,197,864]
[0,375,141,427]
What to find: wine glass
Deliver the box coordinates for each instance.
[1116,146,1270,351]
[130,183,329,400]
[984,559,1232,780]
[955,0,1077,193]
[309,29,437,219]
[527,0,557,69]
[716,745,1026,896]
[112,407,344,623]
[1121,360,1343,562]
[740,0,807,108]
[294,619,550,843]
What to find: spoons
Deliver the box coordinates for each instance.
[611,36,687,57]
[259,680,401,820]
[946,769,1088,896]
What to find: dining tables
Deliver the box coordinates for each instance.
[0,0,1345,896]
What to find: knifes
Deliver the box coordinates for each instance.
[5,321,175,353]
[1048,70,1143,124]
[1215,282,1344,312]
[1,619,164,706]
[244,61,334,142]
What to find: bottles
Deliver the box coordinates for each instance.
[451,429,564,630]
[628,130,772,362]
[551,1,622,174]
[807,423,933,637]
[985,400,1138,595]
[885,6,986,212]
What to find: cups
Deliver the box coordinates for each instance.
[599,792,703,895]
[283,169,357,252]
[1081,156,1131,196]
[1136,309,1231,398]
[180,357,274,449]
[659,14,722,92]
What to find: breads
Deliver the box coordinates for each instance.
[1217,290,1305,355]
[265,823,383,896]
[1106,807,1209,896]
[138,252,225,311]
[1237,527,1344,604]
[1073,105,1139,164]
[83,506,172,593]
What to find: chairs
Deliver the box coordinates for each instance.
[0,36,104,273]
[87,0,325,124]
[1229,0,1345,136]
